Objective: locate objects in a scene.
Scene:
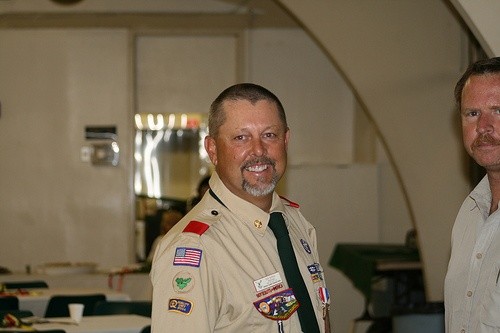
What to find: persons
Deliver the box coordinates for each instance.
[444,56,500,333]
[149,83,333,333]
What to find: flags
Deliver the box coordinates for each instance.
[173,247,202,268]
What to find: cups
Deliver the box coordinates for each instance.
[68,304,85,324]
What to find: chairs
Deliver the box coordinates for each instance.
[0,281,152,317]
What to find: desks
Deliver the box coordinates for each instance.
[0,273,153,301]
[0,288,129,317]
[0,314,151,333]
[328,244,444,333]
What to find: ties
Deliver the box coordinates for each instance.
[209,188,320,333]
